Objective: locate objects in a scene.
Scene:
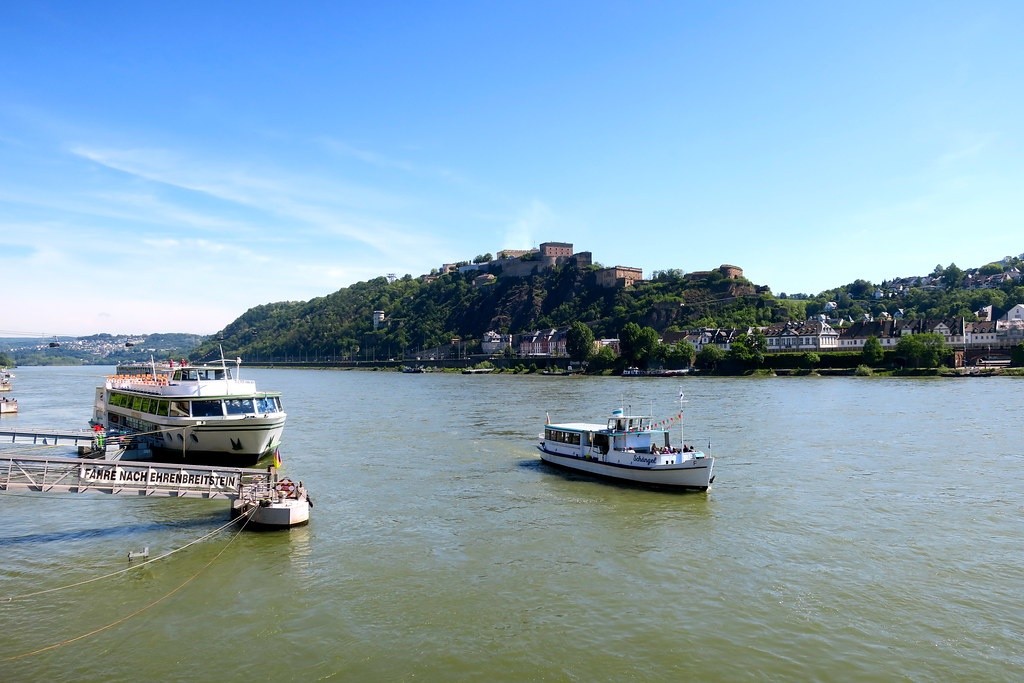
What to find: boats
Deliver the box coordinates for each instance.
[621,367,671,377]
[0,367,15,392]
[537,405,714,493]
[231,478,310,530]
[87,365,287,462]
[116,361,194,376]
[462,369,495,374]
[0,398,19,414]
[542,371,569,376]
[402,368,426,374]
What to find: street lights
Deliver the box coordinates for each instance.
[451,339,460,360]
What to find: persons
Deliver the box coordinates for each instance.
[94,423,104,451]
[170,358,173,367]
[181,357,190,367]
[622,447,635,453]
[615,419,624,430]
[651,443,695,455]
